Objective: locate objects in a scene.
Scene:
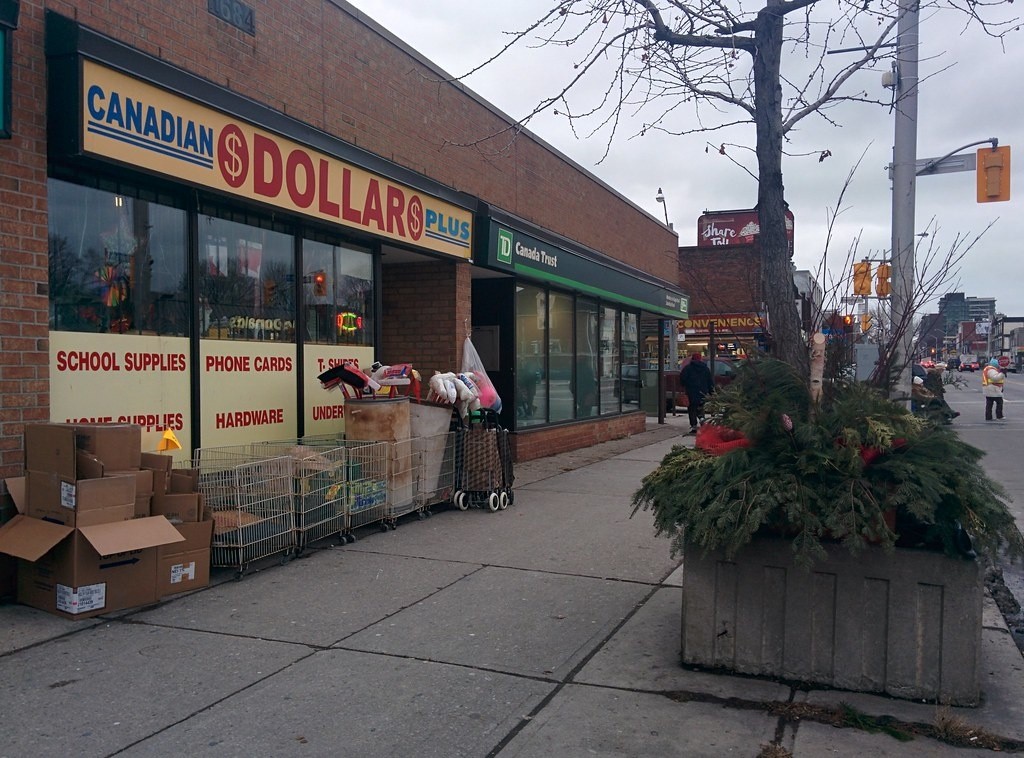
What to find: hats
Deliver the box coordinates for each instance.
[692,353,702,360]
[989,358,1000,368]
[935,364,946,370]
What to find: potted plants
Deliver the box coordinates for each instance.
[628,354,1024,707]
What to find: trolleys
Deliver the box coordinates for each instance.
[168,432,458,582]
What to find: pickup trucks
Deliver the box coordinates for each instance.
[665,355,746,411]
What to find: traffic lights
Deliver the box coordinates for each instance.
[843,315,853,333]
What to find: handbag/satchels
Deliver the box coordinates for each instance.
[676,387,690,407]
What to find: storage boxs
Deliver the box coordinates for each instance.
[0,422,215,621]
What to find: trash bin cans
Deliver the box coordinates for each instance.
[640,368,660,417]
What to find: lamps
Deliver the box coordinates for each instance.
[656,188,664,202]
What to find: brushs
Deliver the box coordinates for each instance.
[318,359,412,400]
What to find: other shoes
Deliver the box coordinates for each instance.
[689,429,697,434]
[952,412,960,418]
[700,420,706,426]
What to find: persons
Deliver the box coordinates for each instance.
[680,351,714,433]
[982,359,1006,422]
[912,363,960,425]
[518,362,538,419]
[568,356,597,418]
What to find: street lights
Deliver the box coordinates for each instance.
[926,334,937,365]
[881,232,929,346]
[975,307,991,364]
[934,328,948,359]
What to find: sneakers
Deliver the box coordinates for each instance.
[986,418,996,421]
[996,416,1007,422]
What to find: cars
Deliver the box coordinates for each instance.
[1001,362,1016,373]
[920,360,946,367]
[972,361,980,370]
[947,358,961,371]
[613,364,643,404]
[959,362,975,372]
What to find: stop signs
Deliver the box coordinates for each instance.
[998,356,1010,367]
